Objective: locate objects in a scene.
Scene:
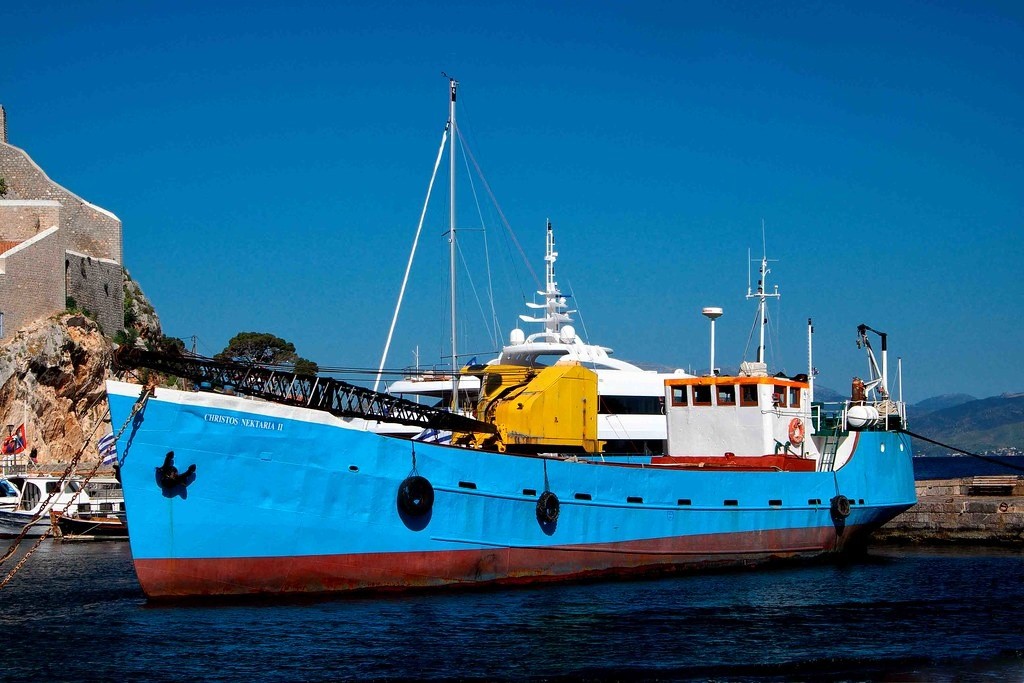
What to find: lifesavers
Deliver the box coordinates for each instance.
[789,418,805,444]
[832,495,850,518]
[536,491,560,523]
[398,476,434,516]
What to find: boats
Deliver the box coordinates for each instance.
[49,479,130,542]
[0,472,124,539]
[105,78,918,605]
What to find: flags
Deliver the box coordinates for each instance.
[97,431,118,465]
[1,424,26,455]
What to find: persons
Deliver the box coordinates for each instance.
[30,445,38,464]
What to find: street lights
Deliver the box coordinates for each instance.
[6,425,14,465]
